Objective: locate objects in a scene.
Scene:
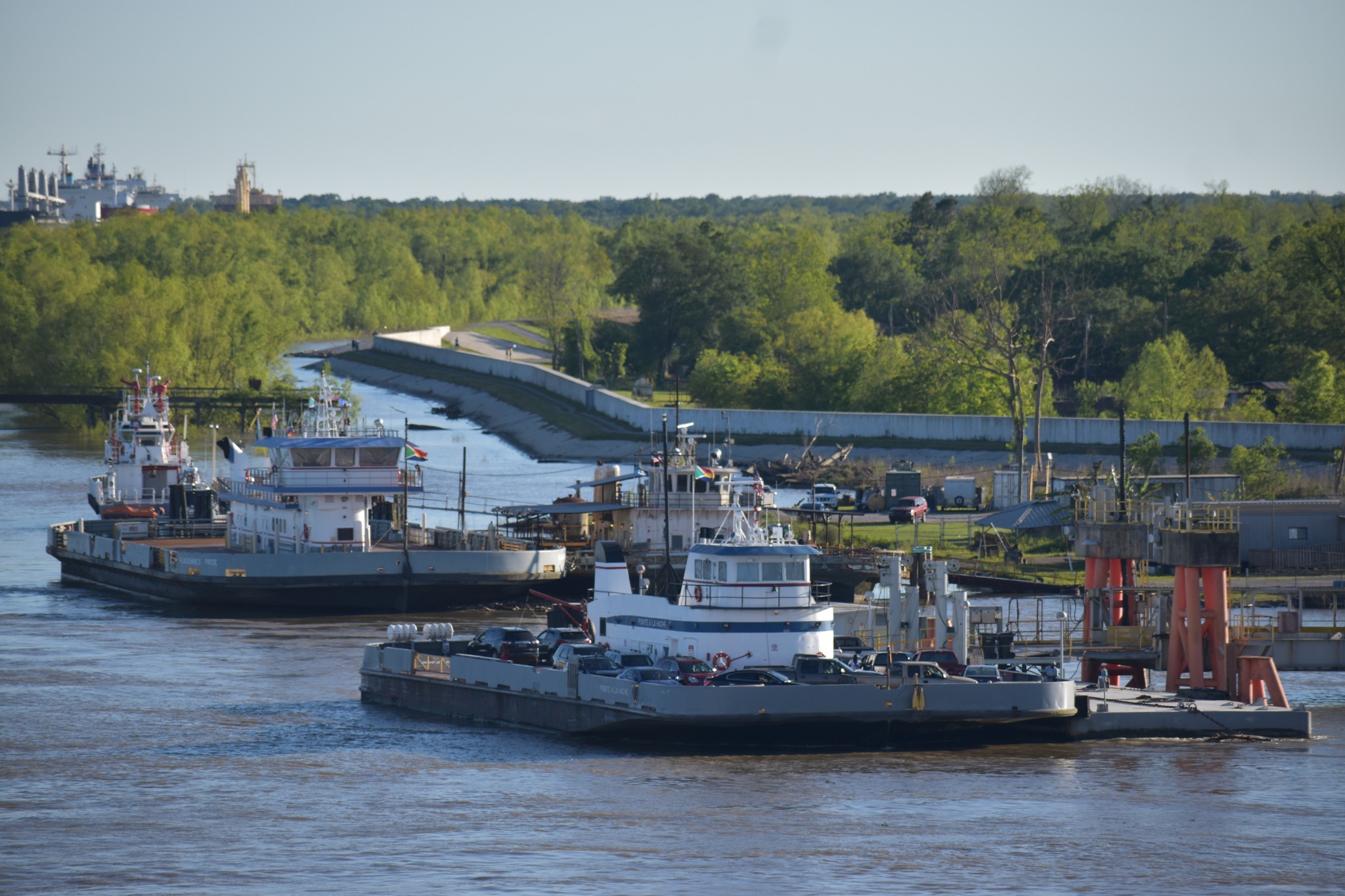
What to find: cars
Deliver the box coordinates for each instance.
[616,666,683,687]
[832,636,876,658]
[962,664,1006,685]
[702,668,805,686]
[565,655,622,678]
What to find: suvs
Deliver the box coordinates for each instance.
[549,643,607,670]
[466,627,539,663]
[888,496,929,524]
[537,627,592,659]
[603,648,655,667]
[806,483,841,512]
[651,655,718,686]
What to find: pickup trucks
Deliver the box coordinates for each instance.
[742,653,883,685]
[912,649,968,676]
[854,661,979,688]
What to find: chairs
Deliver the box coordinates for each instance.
[623,657,634,666]
[664,661,672,671]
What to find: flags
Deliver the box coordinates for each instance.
[653,451,664,463]
[753,465,763,497]
[404,442,428,462]
[285,415,295,437]
[695,465,714,483]
[271,406,278,430]
[308,393,352,410]
[634,464,648,478]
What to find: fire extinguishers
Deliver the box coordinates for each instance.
[707,652,710,660]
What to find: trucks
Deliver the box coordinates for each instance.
[942,474,978,509]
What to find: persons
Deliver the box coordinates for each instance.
[824,661,832,674]
[1040,661,1058,683]
[848,654,861,672]
[304,524,310,542]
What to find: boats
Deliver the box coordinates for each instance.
[359,374,1077,748]
[43,364,571,617]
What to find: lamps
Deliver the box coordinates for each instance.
[374,419,384,429]
[767,586,779,594]
[785,531,794,545]
[710,448,722,467]
[753,527,763,545]
[730,532,740,545]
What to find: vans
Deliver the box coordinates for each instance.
[859,652,909,675]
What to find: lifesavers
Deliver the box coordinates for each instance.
[245,469,250,483]
[694,585,702,602]
[713,653,731,671]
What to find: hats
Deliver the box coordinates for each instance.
[1051,661,1056,665]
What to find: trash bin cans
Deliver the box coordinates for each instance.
[996,632,1016,659]
[978,632,997,660]
[157,516,171,536]
